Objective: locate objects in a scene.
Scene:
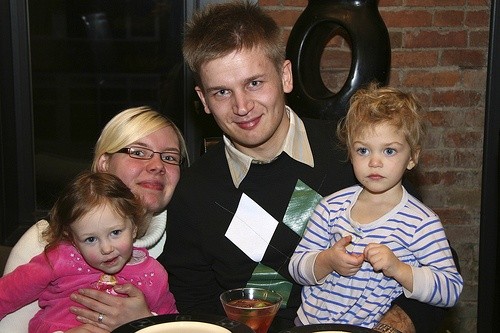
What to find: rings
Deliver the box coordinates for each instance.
[98,313,103,324]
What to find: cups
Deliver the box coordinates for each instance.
[220,288,283,333]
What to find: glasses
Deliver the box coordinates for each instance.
[116,146,183,165]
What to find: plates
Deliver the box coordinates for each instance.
[111,314,254,333]
[284,323,382,333]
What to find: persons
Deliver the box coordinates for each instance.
[156,0,462,333]
[0,171,179,333]
[0,106,190,333]
[288,79,463,333]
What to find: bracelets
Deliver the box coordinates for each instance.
[372,322,402,333]
